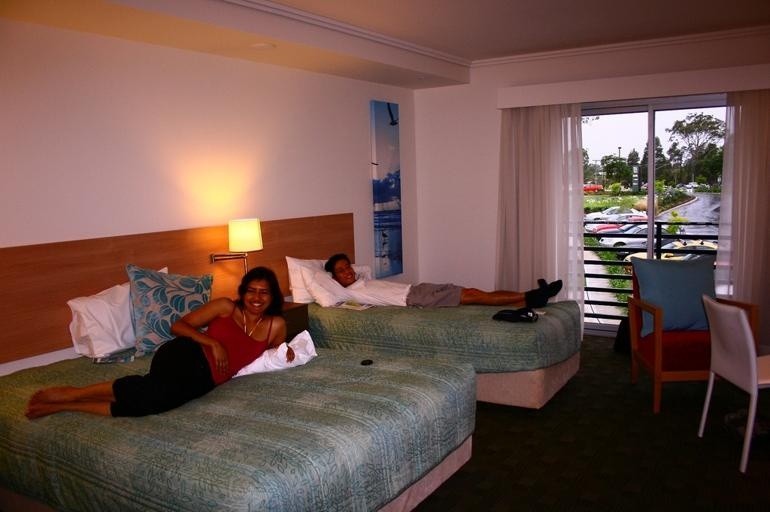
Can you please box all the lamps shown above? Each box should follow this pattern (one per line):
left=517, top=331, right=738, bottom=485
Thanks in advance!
left=210, top=217, right=264, bottom=273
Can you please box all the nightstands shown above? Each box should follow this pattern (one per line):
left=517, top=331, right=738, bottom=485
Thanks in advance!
left=282, top=300, right=309, bottom=341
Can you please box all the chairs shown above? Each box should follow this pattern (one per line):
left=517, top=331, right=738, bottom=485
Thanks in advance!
left=625, top=264, right=770, bottom=472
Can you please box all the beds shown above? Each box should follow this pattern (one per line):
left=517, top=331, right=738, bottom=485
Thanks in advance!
left=0, top=341, right=477, bottom=512
left=284, top=283, right=584, bottom=413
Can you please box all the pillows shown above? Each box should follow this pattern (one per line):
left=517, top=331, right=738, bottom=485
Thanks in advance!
left=630, top=255, right=718, bottom=338
left=67, top=262, right=213, bottom=358
left=283, top=252, right=359, bottom=309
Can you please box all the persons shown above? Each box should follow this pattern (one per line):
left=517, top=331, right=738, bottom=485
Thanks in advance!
left=23, top=266, right=295, bottom=420
left=323, top=249, right=564, bottom=309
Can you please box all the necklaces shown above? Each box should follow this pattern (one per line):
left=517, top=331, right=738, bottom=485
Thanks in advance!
left=243, top=310, right=262, bottom=338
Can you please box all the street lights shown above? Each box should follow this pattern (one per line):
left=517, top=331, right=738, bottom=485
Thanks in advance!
left=618, top=146, right=621, bottom=159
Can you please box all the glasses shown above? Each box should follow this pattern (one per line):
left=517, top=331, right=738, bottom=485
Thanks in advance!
left=527, top=311, right=538, bottom=321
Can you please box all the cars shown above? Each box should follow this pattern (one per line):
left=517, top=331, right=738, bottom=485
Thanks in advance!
left=582, top=180, right=632, bottom=195
left=584, top=206, right=718, bottom=273
left=641, top=181, right=710, bottom=192
